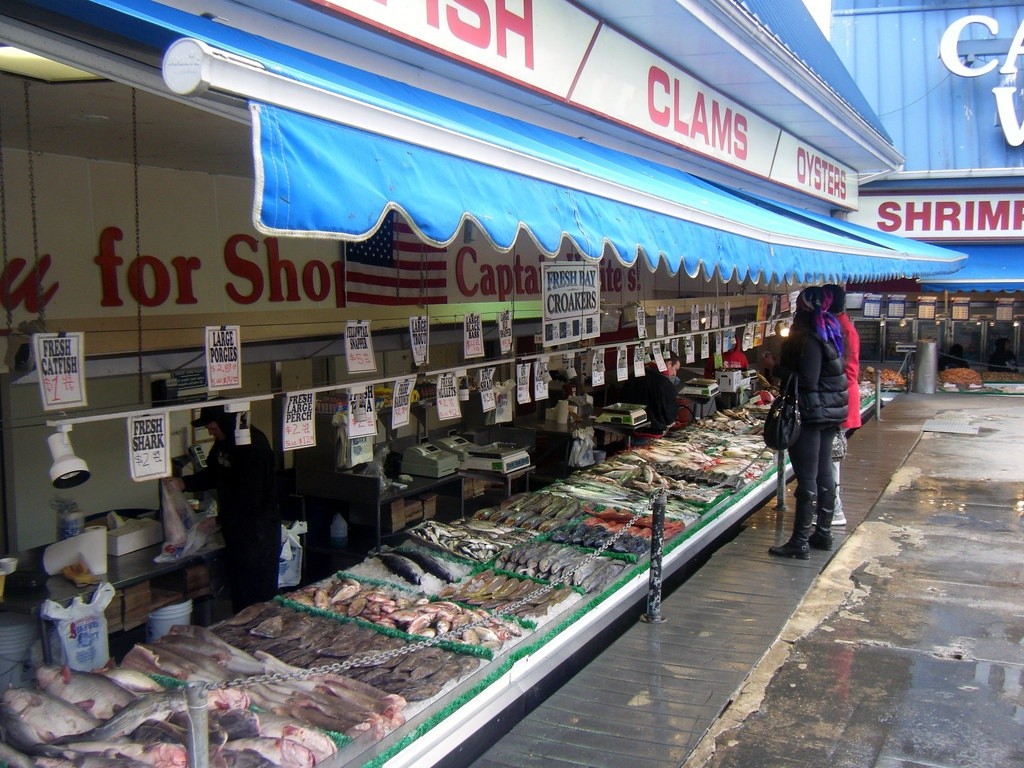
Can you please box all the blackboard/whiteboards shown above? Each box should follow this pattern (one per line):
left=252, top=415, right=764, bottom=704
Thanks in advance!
left=789, top=290, right=799, bottom=313
left=886, top=294, right=907, bottom=318
left=951, top=297, right=970, bottom=321
left=994, top=297, right=1015, bottom=323
left=917, top=296, right=937, bottom=320
left=863, top=294, right=882, bottom=317
left=780, top=294, right=790, bottom=312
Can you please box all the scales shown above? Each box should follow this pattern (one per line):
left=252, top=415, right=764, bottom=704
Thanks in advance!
left=677, top=378, right=720, bottom=397
left=464, top=442, right=531, bottom=474
left=594, top=402, right=649, bottom=426
left=880, top=342, right=917, bottom=393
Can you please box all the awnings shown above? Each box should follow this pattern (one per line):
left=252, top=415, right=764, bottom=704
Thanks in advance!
left=917, top=246, right=1024, bottom=294
left=1, top=1, right=970, bottom=282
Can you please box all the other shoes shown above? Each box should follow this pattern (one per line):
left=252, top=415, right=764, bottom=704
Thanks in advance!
left=812, top=512, right=846, bottom=525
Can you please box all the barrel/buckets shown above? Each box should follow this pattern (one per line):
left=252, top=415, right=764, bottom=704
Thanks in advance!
left=0, top=613, right=38, bottom=696
left=594, top=450, right=606, bottom=464
left=146, top=598, right=193, bottom=643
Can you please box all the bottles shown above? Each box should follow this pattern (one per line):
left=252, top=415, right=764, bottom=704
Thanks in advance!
left=331, top=514, right=347, bottom=547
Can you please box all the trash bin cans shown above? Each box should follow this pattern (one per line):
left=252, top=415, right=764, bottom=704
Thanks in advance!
left=916, top=339, right=938, bottom=395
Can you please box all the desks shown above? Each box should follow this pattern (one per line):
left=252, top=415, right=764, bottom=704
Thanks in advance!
left=329, top=458, right=537, bottom=563
left=498, top=373, right=759, bottom=483
left=20, top=535, right=225, bottom=669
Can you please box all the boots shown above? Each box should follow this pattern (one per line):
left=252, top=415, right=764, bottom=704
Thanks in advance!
left=809, top=483, right=837, bottom=552
left=770, top=485, right=816, bottom=559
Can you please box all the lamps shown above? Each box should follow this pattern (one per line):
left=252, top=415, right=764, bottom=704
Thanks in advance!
left=538, top=357, right=553, bottom=383
left=744, top=325, right=751, bottom=339
left=683, top=336, right=693, bottom=355
left=595, top=349, right=605, bottom=371
left=45, top=424, right=90, bottom=490
left=347, top=387, right=368, bottom=422
left=728, top=328, right=737, bottom=345
left=769, top=323, right=776, bottom=337
left=755, top=325, right=761, bottom=339
left=778, top=320, right=790, bottom=337
left=224, top=401, right=252, bottom=445
left=899, top=320, right=907, bottom=327
left=660, top=340, right=670, bottom=359
left=880, top=321, right=885, bottom=326
left=564, top=353, right=578, bottom=380
left=617, top=345, right=627, bottom=368
left=990, top=321, right=996, bottom=326
left=642, top=342, right=651, bottom=364
left=1013, top=321, right=1019, bottom=327
left=455, top=370, right=469, bottom=401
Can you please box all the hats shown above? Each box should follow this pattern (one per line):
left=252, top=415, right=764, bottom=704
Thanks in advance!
left=796, top=286, right=825, bottom=319
left=822, top=284, right=844, bottom=306
left=191, top=396, right=238, bottom=439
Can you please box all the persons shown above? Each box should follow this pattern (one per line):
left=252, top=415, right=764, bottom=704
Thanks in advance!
left=627, top=351, right=680, bottom=448
left=769, top=290, right=848, bottom=558
left=706, top=338, right=749, bottom=381
left=810, top=284, right=860, bottom=524
left=169, top=398, right=282, bottom=613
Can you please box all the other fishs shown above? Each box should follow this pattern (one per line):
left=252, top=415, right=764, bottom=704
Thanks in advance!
left=162, top=498, right=216, bottom=553
left=344, top=409, right=777, bottom=636
left=0, top=579, right=522, bottom=768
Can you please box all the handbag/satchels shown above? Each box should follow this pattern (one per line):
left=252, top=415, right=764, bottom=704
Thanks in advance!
left=763, top=371, right=801, bottom=450
left=161, top=479, right=199, bottom=546
left=832, top=426, right=848, bottom=462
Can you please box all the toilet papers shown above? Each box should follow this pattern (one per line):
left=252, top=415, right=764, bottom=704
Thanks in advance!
left=557, top=400, right=568, bottom=424
left=43, top=525, right=107, bottom=576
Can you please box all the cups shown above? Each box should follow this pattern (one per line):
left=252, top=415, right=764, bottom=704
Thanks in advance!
left=187, top=499, right=199, bottom=510
left=0, top=569, right=6, bottom=596
left=0, top=558, right=18, bottom=575
